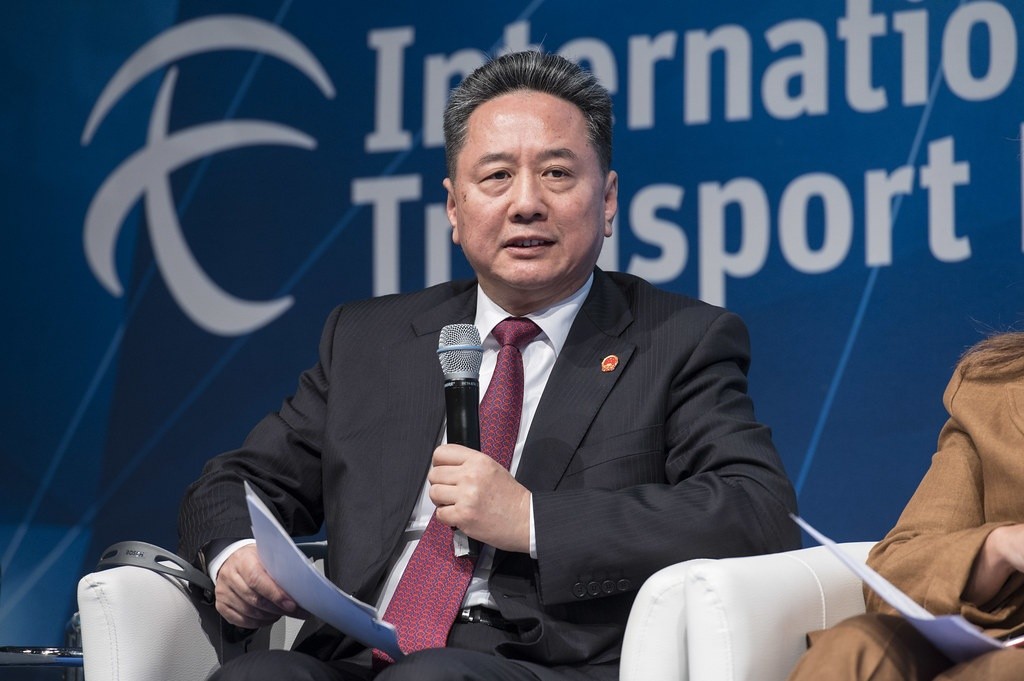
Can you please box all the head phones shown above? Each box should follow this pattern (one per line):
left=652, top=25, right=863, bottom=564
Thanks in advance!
left=96, top=541, right=260, bottom=643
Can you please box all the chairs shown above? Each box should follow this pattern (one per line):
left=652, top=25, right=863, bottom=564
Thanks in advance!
left=78, top=540, right=718, bottom=681
left=684, top=541, right=876, bottom=681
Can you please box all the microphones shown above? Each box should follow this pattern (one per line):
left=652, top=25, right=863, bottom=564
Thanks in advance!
left=438, top=323, right=483, bottom=529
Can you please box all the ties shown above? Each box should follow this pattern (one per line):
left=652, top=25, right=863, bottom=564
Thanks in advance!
left=373, top=316, right=543, bottom=663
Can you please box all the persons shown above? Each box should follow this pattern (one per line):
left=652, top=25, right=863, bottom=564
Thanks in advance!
left=787, top=331, right=1024, bottom=681
left=177, top=50, right=803, bottom=681
